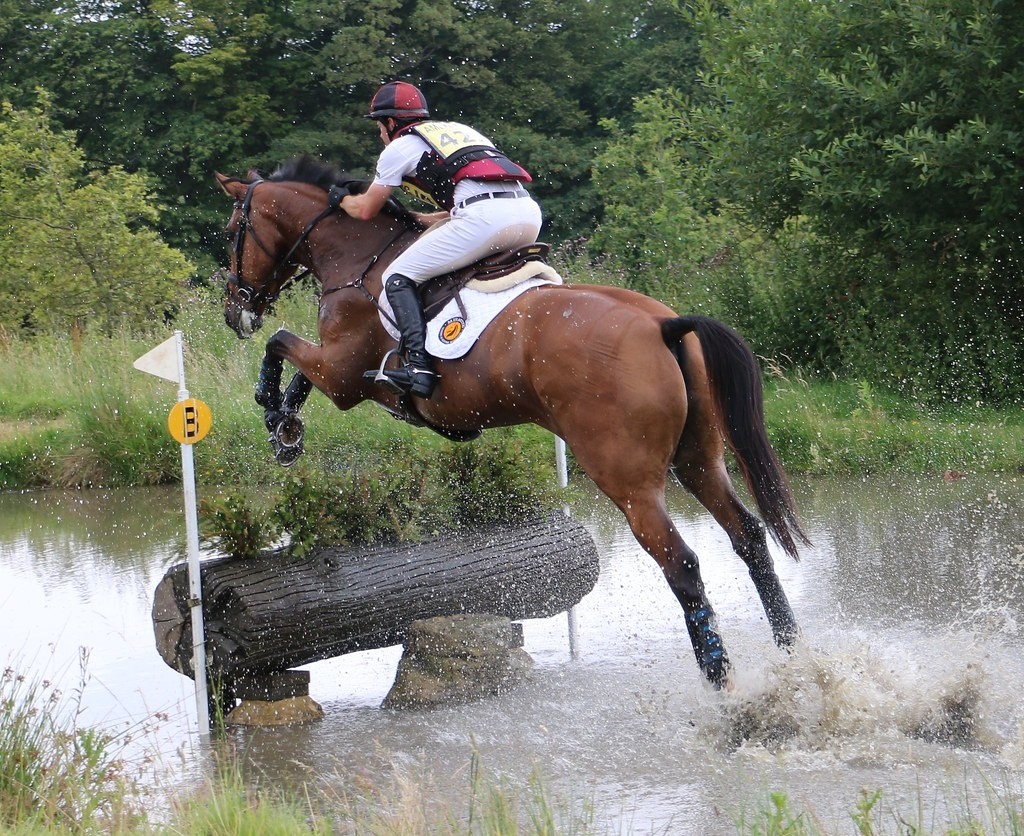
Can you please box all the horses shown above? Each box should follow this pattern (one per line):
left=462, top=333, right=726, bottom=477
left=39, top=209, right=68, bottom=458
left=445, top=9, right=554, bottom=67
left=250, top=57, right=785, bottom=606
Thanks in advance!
left=214, top=154, right=816, bottom=700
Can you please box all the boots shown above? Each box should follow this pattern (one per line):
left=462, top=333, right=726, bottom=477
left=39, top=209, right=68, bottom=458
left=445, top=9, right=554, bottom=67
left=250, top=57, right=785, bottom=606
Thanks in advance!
left=363, top=270, right=435, bottom=398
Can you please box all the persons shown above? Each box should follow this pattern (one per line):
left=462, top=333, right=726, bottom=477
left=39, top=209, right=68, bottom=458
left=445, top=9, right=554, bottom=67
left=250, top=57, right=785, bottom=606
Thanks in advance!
left=327, top=78, right=547, bottom=395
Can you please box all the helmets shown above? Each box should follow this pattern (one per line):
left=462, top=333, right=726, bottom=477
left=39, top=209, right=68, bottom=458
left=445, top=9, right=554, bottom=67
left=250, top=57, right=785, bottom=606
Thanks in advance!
left=363, top=82, right=431, bottom=119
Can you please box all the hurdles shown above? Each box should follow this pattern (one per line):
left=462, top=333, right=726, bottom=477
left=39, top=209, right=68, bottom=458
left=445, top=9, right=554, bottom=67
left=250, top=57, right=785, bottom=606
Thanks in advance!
left=132, top=328, right=602, bottom=735
